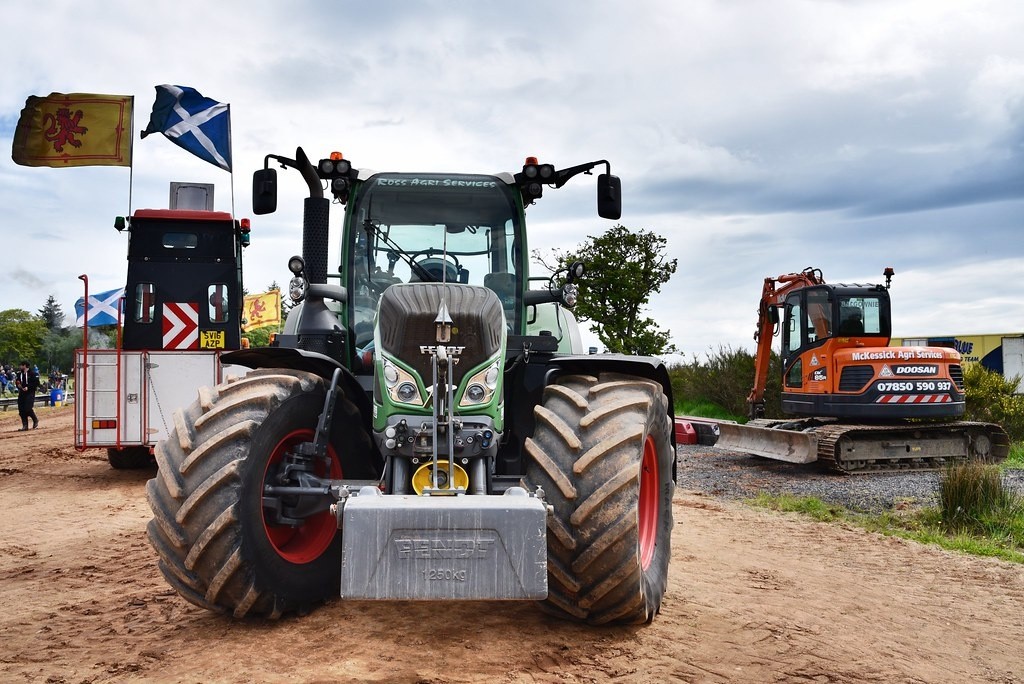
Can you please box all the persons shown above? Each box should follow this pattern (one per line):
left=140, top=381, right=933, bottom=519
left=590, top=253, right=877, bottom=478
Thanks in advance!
left=0, top=363, right=62, bottom=397
left=15, top=360, right=39, bottom=431
left=840, top=308, right=863, bottom=333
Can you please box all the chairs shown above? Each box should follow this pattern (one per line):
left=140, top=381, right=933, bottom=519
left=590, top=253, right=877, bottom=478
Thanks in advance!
left=838, top=306, right=865, bottom=338
left=409, top=248, right=461, bottom=283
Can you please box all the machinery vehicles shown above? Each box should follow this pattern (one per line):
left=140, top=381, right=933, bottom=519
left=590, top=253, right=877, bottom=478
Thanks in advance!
left=708, top=265, right=1012, bottom=478
left=73, top=181, right=250, bottom=470
left=145, top=146, right=720, bottom=630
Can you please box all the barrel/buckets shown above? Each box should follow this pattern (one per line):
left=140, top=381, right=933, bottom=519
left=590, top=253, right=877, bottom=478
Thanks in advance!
left=55, top=401, right=61, bottom=407
left=51, top=388, right=63, bottom=406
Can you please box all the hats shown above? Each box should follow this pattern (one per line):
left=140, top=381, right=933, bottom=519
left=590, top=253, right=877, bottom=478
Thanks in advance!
left=18, top=361, right=30, bottom=368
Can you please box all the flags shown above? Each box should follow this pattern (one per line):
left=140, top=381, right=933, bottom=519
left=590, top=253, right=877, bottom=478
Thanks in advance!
left=75, top=288, right=126, bottom=328
left=138, top=83, right=231, bottom=172
left=12, top=91, right=132, bottom=169
left=240, top=289, right=282, bottom=332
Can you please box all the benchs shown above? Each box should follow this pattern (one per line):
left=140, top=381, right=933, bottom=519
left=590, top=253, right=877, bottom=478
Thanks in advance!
left=0, top=393, right=75, bottom=412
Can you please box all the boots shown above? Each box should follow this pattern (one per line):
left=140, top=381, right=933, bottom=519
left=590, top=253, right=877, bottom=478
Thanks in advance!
left=18, top=421, right=29, bottom=431
left=32, top=417, right=38, bottom=428
